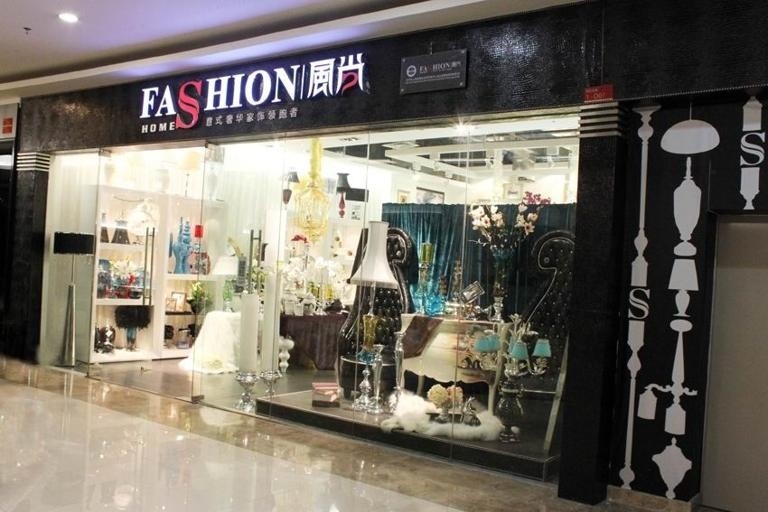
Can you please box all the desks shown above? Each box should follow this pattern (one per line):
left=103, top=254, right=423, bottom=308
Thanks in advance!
left=196, top=308, right=348, bottom=374
left=395, top=310, right=500, bottom=415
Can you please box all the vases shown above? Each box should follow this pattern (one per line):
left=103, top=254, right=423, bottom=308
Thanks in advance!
left=412, top=242, right=448, bottom=314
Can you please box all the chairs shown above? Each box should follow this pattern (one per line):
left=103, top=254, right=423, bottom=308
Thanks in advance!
left=494, top=229, right=575, bottom=401
left=337, top=228, right=414, bottom=402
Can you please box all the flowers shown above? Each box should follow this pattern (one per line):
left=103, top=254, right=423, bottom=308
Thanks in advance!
left=468, top=189, right=551, bottom=297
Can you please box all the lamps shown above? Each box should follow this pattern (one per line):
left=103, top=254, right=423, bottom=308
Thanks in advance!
left=347, top=219, right=401, bottom=361
left=471, top=311, right=552, bottom=442
left=281, top=170, right=299, bottom=206
left=52, top=232, right=96, bottom=368
left=210, top=255, right=241, bottom=312
left=336, top=146, right=351, bottom=216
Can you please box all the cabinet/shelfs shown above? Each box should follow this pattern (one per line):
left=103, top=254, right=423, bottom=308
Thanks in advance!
left=75, top=186, right=231, bottom=363
